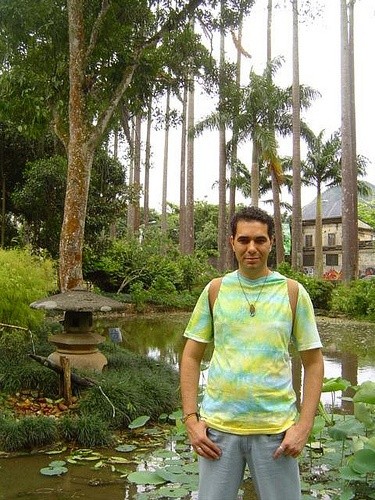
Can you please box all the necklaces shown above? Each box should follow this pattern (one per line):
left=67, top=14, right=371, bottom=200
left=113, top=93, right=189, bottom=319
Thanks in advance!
left=237, top=269, right=269, bottom=318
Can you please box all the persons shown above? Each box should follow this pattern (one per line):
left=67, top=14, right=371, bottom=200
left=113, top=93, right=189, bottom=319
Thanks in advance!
left=181, top=208, right=324, bottom=499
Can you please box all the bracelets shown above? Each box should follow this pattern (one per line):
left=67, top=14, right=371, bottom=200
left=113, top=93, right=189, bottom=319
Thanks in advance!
left=180, top=413, right=198, bottom=424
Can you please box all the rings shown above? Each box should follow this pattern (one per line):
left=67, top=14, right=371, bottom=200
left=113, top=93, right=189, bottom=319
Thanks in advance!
left=196, top=447, right=203, bottom=451
left=278, top=444, right=285, bottom=452
